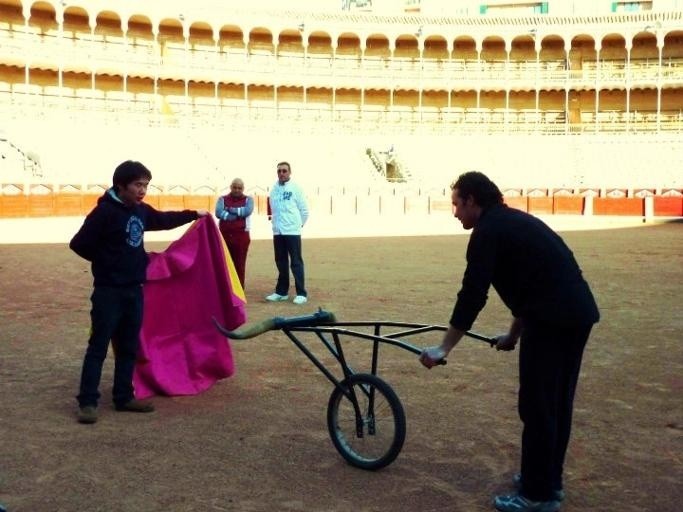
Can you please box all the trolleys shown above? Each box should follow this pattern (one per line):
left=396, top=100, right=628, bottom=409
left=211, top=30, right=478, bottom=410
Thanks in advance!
left=204, top=298, right=514, bottom=472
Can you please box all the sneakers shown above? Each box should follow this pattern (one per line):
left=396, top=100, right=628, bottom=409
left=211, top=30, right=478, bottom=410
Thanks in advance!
left=493, top=491, right=562, bottom=512
left=265, top=292, right=289, bottom=301
left=76, top=403, right=99, bottom=424
left=512, top=472, right=566, bottom=500
left=111, top=394, right=155, bottom=413
left=292, top=295, right=307, bottom=304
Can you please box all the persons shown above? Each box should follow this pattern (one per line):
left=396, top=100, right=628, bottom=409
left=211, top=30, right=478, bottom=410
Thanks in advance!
left=213, top=177, right=255, bottom=290
left=67, top=160, right=210, bottom=424
left=418, top=169, right=601, bottom=512
left=264, top=161, right=309, bottom=305
